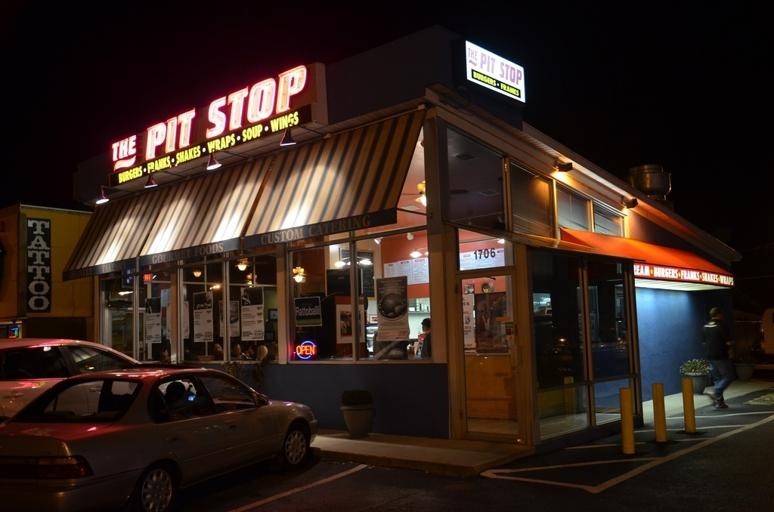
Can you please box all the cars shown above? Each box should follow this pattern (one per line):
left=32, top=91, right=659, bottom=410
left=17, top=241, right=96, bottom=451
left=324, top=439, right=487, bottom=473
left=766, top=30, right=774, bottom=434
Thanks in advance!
left=1, top=364, right=320, bottom=510
left=0, top=337, right=167, bottom=429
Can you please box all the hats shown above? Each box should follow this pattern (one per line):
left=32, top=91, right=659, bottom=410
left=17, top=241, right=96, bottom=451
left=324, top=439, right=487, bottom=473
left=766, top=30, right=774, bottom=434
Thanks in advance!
left=421, top=318, right=430, bottom=326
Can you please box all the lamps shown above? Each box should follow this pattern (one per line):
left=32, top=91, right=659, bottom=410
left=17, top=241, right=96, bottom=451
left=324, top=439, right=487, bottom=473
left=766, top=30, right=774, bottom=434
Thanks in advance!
left=92, top=186, right=112, bottom=208
left=373, top=232, right=415, bottom=245
left=621, top=196, right=638, bottom=208
left=141, top=165, right=163, bottom=193
left=552, top=159, right=572, bottom=172
left=236, top=255, right=249, bottom=272
left=279, top=129, right=298, bottom=149
left=205, top=148, right=225, bottom=173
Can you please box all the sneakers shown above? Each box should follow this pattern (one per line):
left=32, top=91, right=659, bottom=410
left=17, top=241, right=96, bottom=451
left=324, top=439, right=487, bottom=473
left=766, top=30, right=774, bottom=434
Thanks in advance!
left=709, top=393, right=728, bottom=408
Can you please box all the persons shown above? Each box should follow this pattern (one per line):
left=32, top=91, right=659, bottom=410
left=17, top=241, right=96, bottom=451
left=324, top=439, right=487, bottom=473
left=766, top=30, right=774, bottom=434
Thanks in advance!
left=183, top=338, right=198, bottom=361
left=415, top=317, right=430, bottom=358
left=165, top=381, right=187, bottom=405
left=230, top=342, right=243, bottom=359
left=212, top=343, right=222, bottom=355
left=701, top=307, right=736, bottom=408
left=240, top=343, right=256, bottom=360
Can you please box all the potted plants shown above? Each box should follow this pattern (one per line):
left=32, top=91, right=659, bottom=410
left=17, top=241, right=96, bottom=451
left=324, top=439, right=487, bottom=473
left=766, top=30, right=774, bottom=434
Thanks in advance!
left=680, top=358, right=713, bottom=394
left=340, top=388, right=377, bottom=435
left=733, top=350, right=758, bottom=380
left=212, top=359, right=265, bottom=404
